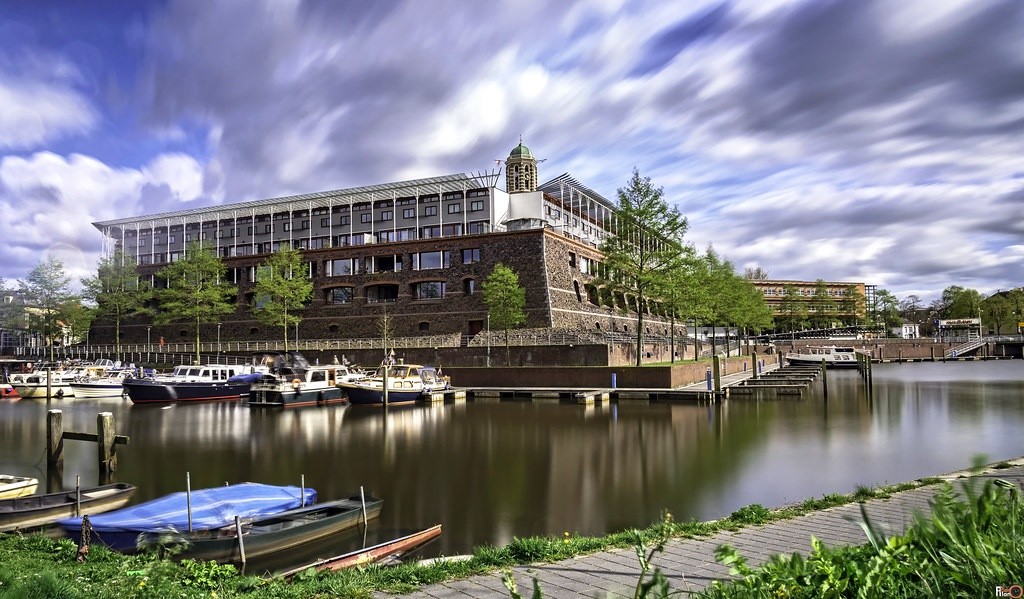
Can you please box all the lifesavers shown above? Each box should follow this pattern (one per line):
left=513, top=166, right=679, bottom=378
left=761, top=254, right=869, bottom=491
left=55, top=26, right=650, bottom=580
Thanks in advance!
left=291, top=379, right=301, bottom=389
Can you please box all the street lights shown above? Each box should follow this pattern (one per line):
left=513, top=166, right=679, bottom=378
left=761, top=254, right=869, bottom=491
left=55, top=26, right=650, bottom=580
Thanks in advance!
left=217, top=323, right=221, bottom=363
left=294, top=321, right=300, bottom=352
left=86, top=328, right=91, bottom=359
left=147, top=326, right=151, bottom=362
left=487, top=315, right=490, bottom=366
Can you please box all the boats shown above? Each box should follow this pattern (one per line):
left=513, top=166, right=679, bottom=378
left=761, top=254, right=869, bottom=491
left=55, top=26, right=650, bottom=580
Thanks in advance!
left=336, top=365, right=450, bottom=404
left=0, top=482, right=136, bottom=532
left=0, top=359, right=138, bottom=398
left=249, top=365, right=375, bottom=405
left=57, top=482, right=317, bottom=554
left=785, top=346, right=882, bottom=364
left=135, top=495, right=383, bottom=565
left=121, top=364, right=269, bottom=403
left=0, top=476, right=39, bottom=500
